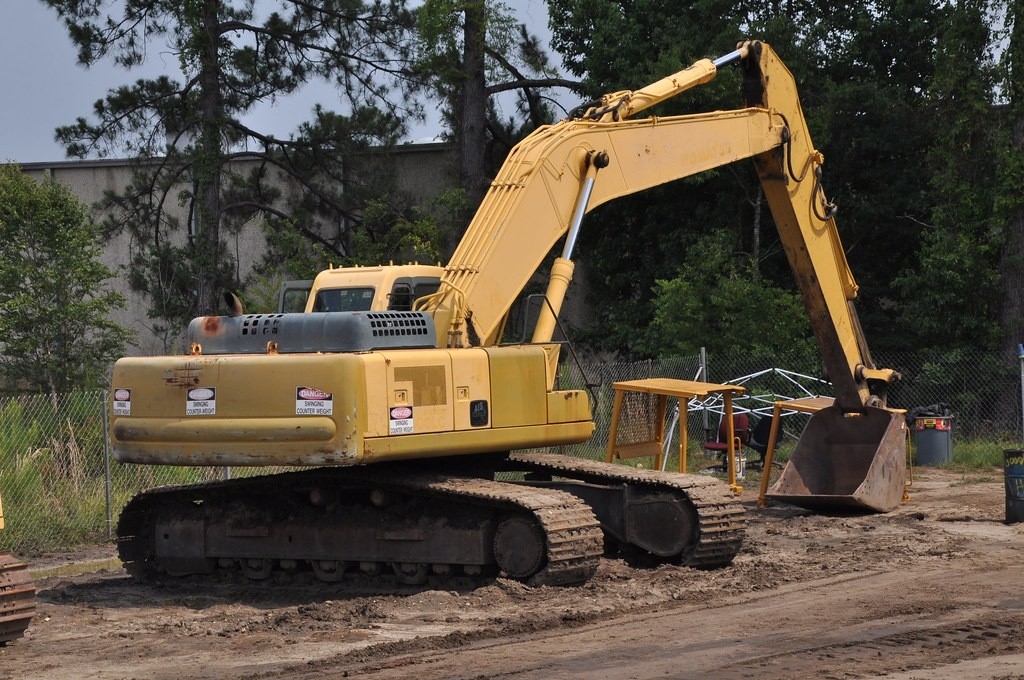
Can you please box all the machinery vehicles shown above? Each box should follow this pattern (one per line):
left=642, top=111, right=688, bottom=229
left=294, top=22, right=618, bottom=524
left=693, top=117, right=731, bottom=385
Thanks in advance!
left=109, top=38, right=911, bottom=590
left=0, top=491, right=37, bottom=646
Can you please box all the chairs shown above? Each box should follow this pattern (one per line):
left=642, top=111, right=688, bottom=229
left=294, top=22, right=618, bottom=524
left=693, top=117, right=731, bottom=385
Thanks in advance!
left=704, top=414, right=749, bottom=473
left=745, top=413, right=786, bottom=471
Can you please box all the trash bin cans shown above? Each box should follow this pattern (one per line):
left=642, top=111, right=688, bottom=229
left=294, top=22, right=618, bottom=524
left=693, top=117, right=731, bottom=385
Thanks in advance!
left=915, top=414, right=954, bottom=466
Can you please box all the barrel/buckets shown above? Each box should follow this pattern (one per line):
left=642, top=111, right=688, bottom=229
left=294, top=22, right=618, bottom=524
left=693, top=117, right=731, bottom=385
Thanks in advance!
left=914, top=416, right=954, bottom=467
left=1002, top=449, right=1024, bottom=525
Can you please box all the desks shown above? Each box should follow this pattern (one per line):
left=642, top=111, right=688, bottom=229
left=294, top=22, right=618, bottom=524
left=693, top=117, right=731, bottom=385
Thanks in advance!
left=605, top=378, right=743, bottom=495
left=757, top=398, right=835, bottom=508
